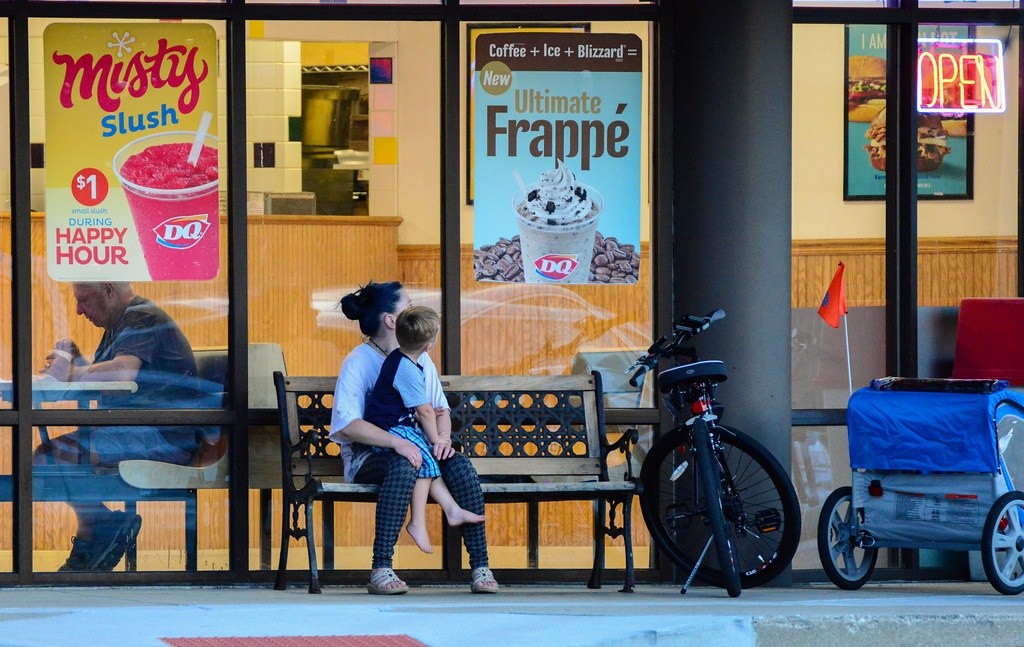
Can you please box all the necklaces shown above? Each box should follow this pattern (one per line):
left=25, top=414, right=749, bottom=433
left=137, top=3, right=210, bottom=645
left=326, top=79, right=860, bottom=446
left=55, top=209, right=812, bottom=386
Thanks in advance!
left=369, top=338, right=388, bottom=357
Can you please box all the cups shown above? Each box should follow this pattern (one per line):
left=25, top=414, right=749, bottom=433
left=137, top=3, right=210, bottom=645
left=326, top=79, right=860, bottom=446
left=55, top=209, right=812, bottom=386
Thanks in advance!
left=111, top=130, right=220, bottom=280
left=53, top=349, right=74, bottom=362
left=511, top=183, right=605, bottom=283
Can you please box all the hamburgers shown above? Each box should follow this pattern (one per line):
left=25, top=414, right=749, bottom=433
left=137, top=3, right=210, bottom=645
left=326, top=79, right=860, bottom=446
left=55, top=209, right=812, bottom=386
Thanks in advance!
left=847, top=55, right=968, bottom=172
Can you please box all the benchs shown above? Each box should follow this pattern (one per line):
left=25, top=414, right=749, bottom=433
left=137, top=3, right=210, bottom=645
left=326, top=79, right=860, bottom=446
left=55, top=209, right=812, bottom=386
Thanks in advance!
left=273, top=369, right=643, bottom=594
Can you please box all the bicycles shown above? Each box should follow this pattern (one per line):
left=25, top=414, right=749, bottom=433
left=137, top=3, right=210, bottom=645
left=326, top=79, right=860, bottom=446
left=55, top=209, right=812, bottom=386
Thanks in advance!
left=623, top=310, right=803, bottom=598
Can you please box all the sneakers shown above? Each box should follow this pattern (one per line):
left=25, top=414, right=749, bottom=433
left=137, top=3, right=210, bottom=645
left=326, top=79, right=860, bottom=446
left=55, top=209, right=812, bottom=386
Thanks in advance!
left=58, top=536, right=95, bottom=571
left=90, top=510, right=142, bottom=572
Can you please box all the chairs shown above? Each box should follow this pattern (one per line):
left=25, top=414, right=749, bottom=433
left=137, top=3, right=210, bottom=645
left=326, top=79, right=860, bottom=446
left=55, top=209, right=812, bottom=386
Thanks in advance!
left=93, top=343, right=287, bottom=571
left=527, top=350, right=663, bottom=568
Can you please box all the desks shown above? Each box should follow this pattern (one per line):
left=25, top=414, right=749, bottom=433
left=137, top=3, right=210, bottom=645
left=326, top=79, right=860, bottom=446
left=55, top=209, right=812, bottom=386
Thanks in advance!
left=0, top=381, right=138, bottom=501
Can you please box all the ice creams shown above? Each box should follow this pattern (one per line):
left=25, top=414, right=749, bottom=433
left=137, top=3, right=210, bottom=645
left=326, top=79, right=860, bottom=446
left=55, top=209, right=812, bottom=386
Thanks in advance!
left=525, top=158, right=593, bottom=225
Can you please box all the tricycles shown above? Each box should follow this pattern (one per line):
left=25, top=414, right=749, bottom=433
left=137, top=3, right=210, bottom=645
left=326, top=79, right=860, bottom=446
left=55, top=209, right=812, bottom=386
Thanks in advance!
left=816, top=376, right=1024, bottom=596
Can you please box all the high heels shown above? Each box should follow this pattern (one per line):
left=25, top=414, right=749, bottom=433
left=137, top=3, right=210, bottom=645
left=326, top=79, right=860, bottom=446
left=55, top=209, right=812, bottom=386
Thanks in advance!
left=366, top=569, right=409, bottom=595
left=470, top=567, right=500, bottom=595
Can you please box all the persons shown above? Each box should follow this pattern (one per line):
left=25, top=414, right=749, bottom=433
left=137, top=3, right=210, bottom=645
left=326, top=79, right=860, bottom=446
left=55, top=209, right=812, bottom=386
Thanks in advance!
left=364, top=306, right=485, bottom=554
left=329, top=278, right=498, bottom=595
left=33, top=282, right=205, bottom=571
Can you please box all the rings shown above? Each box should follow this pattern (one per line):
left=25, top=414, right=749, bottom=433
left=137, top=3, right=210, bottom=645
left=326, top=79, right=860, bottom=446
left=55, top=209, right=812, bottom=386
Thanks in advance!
left=47, top=363, right=49, bottom=368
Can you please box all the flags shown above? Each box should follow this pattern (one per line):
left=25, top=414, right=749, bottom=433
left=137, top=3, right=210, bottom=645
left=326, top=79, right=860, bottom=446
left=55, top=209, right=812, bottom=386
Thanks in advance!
left=817, top=261, right=848, bottom=328
